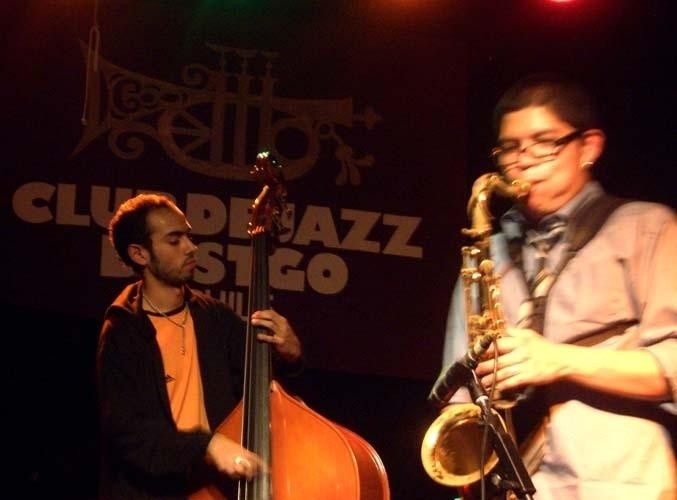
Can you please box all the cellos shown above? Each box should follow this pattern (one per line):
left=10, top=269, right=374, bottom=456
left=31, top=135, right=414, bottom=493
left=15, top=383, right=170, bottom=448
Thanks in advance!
left=187, top=150, right=391, bottom=499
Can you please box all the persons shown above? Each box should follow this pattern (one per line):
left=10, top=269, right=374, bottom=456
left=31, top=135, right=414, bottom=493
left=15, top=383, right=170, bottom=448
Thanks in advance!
left=94, top=191, right=303, bottom=499
left=436, top=72, right=676, bottom=499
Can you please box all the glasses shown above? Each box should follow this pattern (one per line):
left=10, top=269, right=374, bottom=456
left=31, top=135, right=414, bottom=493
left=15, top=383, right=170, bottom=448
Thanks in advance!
left=491, top=131, right=581, bottom=166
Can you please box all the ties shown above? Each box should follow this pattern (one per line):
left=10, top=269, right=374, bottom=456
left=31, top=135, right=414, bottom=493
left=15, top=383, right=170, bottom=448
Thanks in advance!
left=523, top=220, right=565, bottom=334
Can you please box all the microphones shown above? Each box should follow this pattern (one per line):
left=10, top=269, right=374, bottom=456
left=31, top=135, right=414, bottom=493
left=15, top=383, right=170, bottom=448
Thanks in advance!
left=425, top=330, right=500, bottom=413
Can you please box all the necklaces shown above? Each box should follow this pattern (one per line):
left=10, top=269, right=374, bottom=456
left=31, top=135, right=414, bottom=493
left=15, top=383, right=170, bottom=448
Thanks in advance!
left=141, top=293, right=190, bottom=358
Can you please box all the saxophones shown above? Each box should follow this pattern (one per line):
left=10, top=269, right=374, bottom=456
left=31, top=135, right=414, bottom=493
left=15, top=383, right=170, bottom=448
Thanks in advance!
left=420, top=172, right=531, bottom=500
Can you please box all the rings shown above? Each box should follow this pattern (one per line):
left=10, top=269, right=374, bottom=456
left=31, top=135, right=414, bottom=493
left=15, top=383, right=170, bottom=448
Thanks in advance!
left=232, top=456, right=241, bottom=463
